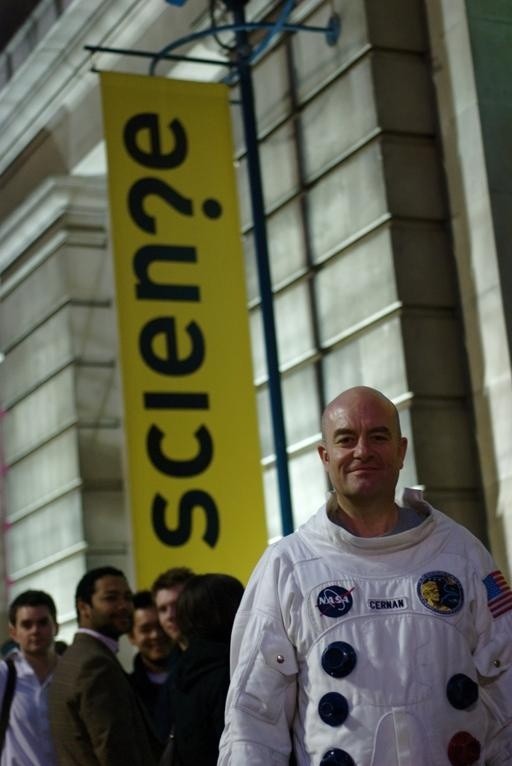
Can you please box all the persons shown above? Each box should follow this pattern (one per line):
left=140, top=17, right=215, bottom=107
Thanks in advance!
left=47, top=564, right=162, bottom=764
left=1, top=589, right=73, bottom=765
left=217, top=384, right=511, bottom=766
left=127, top=567, right=246, bottom=765
